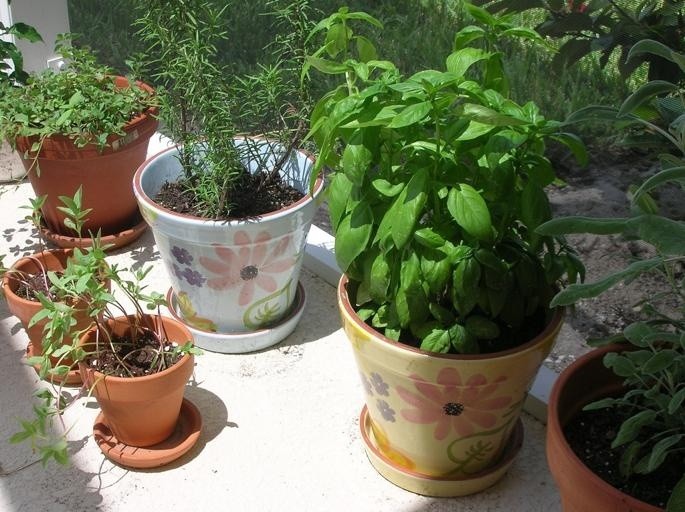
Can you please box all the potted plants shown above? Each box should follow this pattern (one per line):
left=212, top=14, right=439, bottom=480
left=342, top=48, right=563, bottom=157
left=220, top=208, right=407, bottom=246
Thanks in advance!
left=540, top=29, right=684, bottom=512
left=299, top=1, right=587, bottom=502
left=1, top=28, right=162, bottom=254
left=0, top=229, right=204, bottom=471
left=2, top=182, right=114, bottom=387
left=125, top=2, right=327, bottom=358
